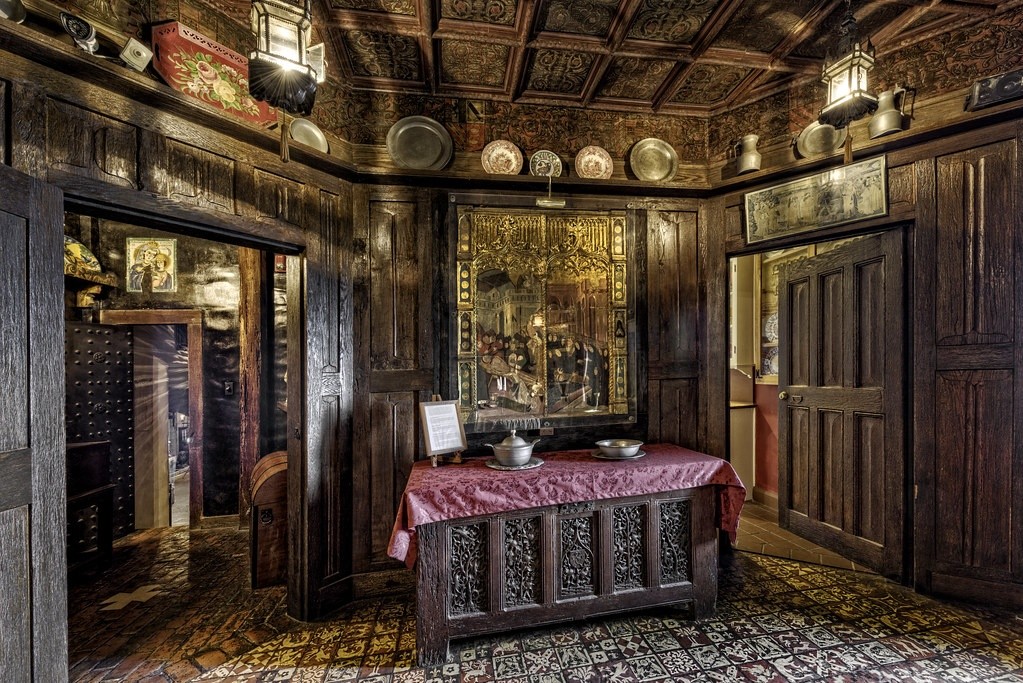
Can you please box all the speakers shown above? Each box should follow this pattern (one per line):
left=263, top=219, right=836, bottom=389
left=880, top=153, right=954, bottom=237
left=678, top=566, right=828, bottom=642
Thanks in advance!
left=963, top=67, right=1023, bottom=112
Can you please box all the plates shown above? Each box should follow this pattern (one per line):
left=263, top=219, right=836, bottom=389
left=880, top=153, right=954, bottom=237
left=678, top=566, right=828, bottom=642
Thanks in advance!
left=630, top=138, right=681, bottom=181
left=575, top=146, right=614, bottom=178
left=387, top=115, right=454, bottom=168
left=484, top=456, right=544, bottom=470
left=592, top=449, right=647, bottom=459
left=289, top=118, right=329, bottom=154
left=481, top=140, right=524, bottom=176
left=530, top=151, right=562, bottom=176
left=797, top=120, right=848, bottom=158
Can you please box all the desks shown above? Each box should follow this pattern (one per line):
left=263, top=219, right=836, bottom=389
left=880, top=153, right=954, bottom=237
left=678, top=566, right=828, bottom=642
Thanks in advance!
left=385, top=442, right=747, bottom=669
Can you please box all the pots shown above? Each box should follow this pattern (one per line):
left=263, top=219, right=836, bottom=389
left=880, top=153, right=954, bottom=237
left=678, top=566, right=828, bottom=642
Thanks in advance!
left=484, top=430, right=541, bottom=465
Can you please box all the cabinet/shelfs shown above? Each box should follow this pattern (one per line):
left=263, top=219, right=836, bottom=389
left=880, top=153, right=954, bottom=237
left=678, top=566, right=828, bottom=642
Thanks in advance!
left=66, top=441, right=117, bottom=588
left=753, top=246, right=815, bottom=385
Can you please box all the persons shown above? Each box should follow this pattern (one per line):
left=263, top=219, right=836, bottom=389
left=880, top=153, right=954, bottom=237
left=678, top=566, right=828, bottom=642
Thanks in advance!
left=471, top=315, right=608, bottom=412
left=130, top=241, right=172, bottom=290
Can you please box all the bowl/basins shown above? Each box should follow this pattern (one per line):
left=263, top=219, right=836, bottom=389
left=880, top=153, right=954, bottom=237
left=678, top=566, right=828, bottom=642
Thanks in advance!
left=595, top=439, right=644, bottom=456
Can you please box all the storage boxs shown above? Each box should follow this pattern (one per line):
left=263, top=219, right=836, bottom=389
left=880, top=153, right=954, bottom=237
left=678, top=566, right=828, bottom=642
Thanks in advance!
left=249, top=452, right=288, bottom=589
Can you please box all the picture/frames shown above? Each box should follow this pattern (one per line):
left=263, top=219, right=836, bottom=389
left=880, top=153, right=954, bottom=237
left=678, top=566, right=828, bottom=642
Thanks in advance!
left=125, top=237, right=178, bottom=293
left=743, top=153, right=889, bottom=247
left=449, top=194, right=638, bottom=435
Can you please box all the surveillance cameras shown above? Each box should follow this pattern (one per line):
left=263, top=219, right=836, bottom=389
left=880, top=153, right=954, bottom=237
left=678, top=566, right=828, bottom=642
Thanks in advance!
left=119, top=36, right=153, bottom=73
left=60, top=12, right=100, bottom=53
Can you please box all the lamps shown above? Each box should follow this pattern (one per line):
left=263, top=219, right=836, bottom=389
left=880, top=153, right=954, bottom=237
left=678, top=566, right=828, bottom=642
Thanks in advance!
left=247, top=1, right=320, bottom=165
left=817, top=2, right=880, bottom=167
left=535, top=176, right=566, bottom=210
